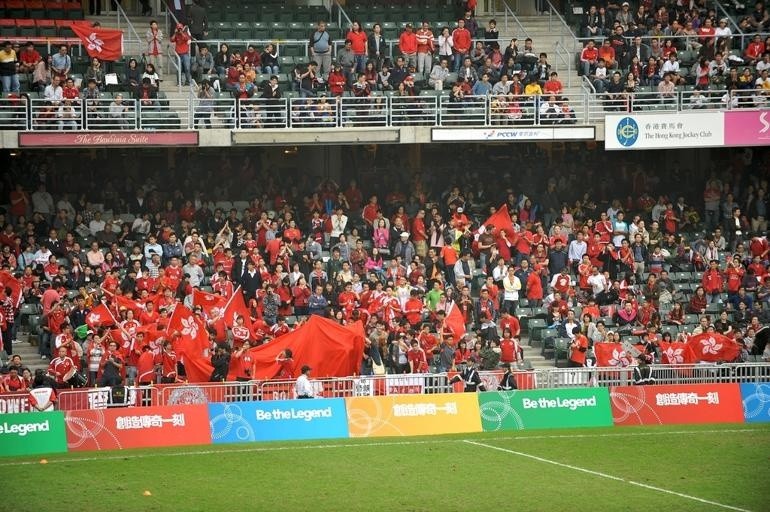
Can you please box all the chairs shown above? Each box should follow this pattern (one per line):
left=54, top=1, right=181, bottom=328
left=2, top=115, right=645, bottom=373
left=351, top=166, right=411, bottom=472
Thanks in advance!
left=511, top=293, right=641, bottom=366
left=1, top=3, right=147, bottom=80
left=180, top=0, right=474, bottom=88
left=647, top=258, right=737, bottom=343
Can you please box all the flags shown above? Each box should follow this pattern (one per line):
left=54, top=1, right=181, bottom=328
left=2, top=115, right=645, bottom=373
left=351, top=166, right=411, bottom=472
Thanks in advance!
left=71, top=22, right=123, bottom=59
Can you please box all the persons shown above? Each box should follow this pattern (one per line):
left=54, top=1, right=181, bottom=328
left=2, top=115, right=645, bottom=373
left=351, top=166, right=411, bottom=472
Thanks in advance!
left=578, top=0, right=769, bottom=112
left=171, top=1, right=578, bottom=124
left=2, top=150, right=769, bottom=411
left=0, top=21, right=163, bottom=128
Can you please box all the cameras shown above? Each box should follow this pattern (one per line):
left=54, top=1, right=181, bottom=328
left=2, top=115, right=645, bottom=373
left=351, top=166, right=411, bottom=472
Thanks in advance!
left=178, top=29, right=180, bottom=32
left=210, top=347, right=218, bottom=352
left=268, top=290, right=271, bottom=295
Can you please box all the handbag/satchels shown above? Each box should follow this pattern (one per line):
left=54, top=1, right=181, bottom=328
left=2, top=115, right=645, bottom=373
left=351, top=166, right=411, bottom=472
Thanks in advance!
left=371, top=359, right=386, bottom=375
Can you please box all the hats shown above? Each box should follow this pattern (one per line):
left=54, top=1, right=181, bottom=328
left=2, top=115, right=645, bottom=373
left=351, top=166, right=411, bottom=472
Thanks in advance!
left=499, top=363, right=511, bottom=368
left=622, top=1, right=630, bottom=7
left=533, top=264, right=545, bottom=270
left=636, top=353, right=646, bottom=361
left=466, top=356, right=475, bottom=362
left=456, top=206, right=465, bottom=214
left=606, top=330, right=615, bottom=336
left=405, top=22, right=413, bottom=28
left=301, top=365, right=312, bottom=371
left=718, top=18, right=728, bottom=24
